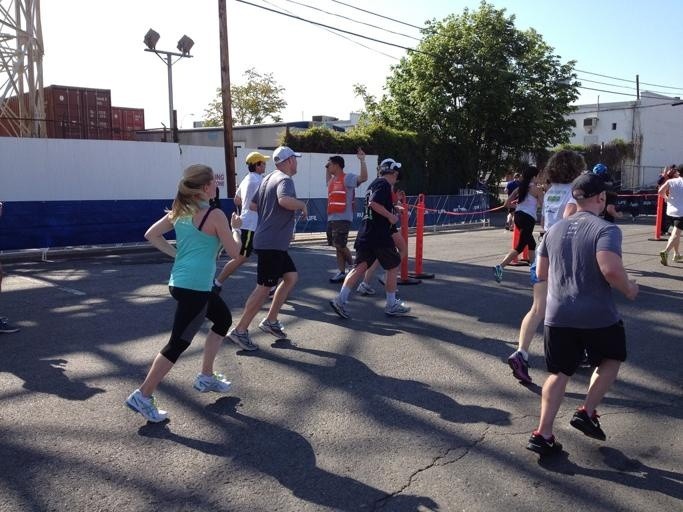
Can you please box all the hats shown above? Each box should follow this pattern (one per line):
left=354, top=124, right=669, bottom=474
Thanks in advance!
left=570, top=174, right=606, bottom=200
left=269, top=147, right=301, bottom=166
left=244, top=153, right=270, bottom=167
left=592, top=163, right=610, bottom=177
left=376, top=157, right=403, bottom=178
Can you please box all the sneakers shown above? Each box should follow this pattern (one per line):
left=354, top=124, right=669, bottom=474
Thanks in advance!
left=672, top=254, right=683, bottom=262
left=507, top=350, right=532, bottom=386
left=525, top=430, right=563, bottom=457
left=259, top=317, right=287, bottom=339
left=356, top=282, right=376, bottom=297
left=268, top=285, right=278, bottom=300
left=384, top=299, right=411, bottom=316
left=123, top=388, right=168, bottom=423
left=330, top=271, right=347, bottom=284
left=211, top=278, right=222, bottom=296
left=492, top=263, right=503, bottom=283
left=660, top=251, right=670, bottom=267
left=226, top=327, right=259, bottom=352
left=191, top=371, right=232, bottom=394
left=328, top=295, right=352, bottom=320
left=0, top=315, right=22, bottom=334
left=570, top=406, right=607, bottom=441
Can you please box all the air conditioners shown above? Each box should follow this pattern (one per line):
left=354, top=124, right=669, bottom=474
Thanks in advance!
left=582, top=118, right=596, bottom=128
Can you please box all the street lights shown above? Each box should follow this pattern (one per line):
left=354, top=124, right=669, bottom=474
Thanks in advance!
left=142, top=28, right=195, bottom=141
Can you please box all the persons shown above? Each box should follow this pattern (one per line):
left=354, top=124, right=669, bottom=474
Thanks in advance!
left=592, top=164, right=624, bottom=224
left=525, top=171, right=640, bottom=455
left=492, top=166, right=546, bottom=283
left=325, top=148, right=367, bottom=284
left=212, top=151, right=279, bottom=300
left=329, top=161, right=411, bottom=319
left=503, top=173, right=520, bottom=231
left=507, top=150, right=591, bottom=384
left=356, top=158, right=406, bottom=294
left=658, top=164, right=683, bottom=266
left=123, top=164, right=242, bottom=423
left=656, top=164, right=680, bottom=236
left=226, top=145, right=308, bottom=351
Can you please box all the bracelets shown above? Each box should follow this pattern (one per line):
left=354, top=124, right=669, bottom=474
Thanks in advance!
left=231, top=229, right=242, bottom=236
